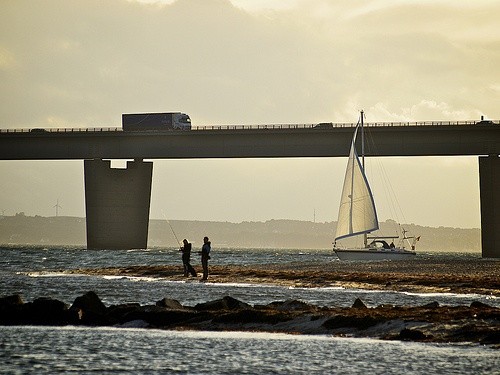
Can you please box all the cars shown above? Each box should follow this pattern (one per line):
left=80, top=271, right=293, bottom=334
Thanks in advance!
left=314, top=123, right=333, bottom=127
left=31, top=128, right=47, bottom=132
left=476, top=121, right=494, bottom=124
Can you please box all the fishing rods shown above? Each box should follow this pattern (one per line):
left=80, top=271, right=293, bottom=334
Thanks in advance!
left=166, top=218, right=184, bottom=253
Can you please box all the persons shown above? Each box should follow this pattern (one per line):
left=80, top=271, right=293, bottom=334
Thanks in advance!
left=390, top=242, right=395, bottom=248
left=182, top=239, right=192, bottom=277
left=201, top=237, right=211, bottom=280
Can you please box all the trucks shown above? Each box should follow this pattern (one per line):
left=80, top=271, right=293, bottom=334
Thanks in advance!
left=122, top=112, right=192, bottom=130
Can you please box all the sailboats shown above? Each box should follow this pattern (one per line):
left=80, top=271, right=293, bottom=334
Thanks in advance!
left=332, top=109, right=423, bottom=263
